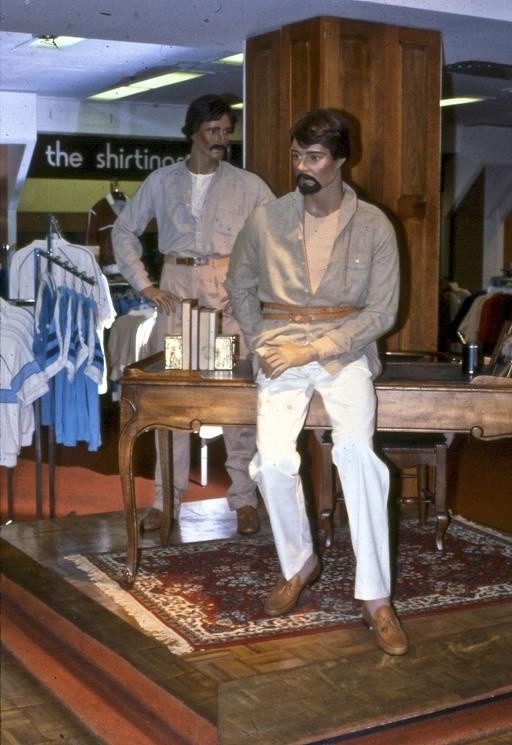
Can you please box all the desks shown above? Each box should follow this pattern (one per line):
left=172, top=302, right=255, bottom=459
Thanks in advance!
left=111, top=346, right=511, bottom=592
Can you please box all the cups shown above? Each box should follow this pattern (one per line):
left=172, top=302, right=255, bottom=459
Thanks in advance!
left=463, top=343, right=480, bottom=373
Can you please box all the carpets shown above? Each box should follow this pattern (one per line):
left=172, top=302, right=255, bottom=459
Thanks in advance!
left=62, top=509, right=510, bottom=659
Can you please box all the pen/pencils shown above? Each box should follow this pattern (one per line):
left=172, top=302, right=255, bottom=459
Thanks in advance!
left=456, top=331, right=468, bottom=345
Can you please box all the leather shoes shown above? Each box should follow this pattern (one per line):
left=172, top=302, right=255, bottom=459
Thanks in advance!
left=362, top=602, right=408, bottom=656
left=140, top=507, right=163, bottom=530
left=236, top=505, right=260, bottom=535
left=264, top=556, right=321, bottom=616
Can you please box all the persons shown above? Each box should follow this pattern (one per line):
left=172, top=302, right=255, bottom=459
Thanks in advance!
left=111, top=93, right=278, bottom=537
left=223, top=108, right=409, bottom=657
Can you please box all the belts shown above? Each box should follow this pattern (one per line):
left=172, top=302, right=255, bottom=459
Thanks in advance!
left=164, top=255, right=229, bottom=267
left=262, top=301, right=362, bottom=323
